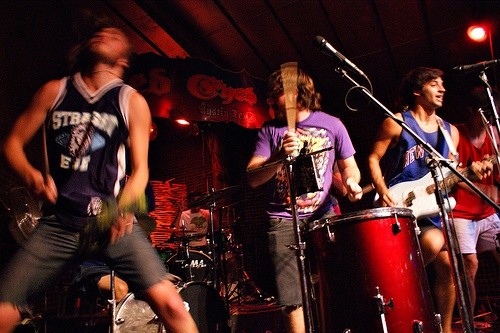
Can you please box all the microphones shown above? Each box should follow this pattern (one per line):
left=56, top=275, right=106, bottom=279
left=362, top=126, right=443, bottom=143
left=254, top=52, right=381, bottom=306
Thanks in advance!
left=453, top=60, right=498, bottom=72
left=314, top=36, right=346, bottom=62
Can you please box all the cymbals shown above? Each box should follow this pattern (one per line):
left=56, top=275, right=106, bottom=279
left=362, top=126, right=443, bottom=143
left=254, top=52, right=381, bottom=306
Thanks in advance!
left=215, top=199, right=249, bottom=213
left=130, top=213, right=157, bottom=236
left=164, top=232, right=203, bottom=244
left=185, top=185, right=241, bottom=208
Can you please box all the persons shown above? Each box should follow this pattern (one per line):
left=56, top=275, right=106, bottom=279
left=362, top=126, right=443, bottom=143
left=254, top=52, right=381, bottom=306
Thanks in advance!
left=239, top=68, right=364, bottom=333
left=64, top=260, right=128, bottom=333
left=177, top=191, right=212, bottom=246
left=0, top=18, right=200, bottom=333
left=367, top=64, right=460, bottom=333
left=452, top=86, right=500, bottom=333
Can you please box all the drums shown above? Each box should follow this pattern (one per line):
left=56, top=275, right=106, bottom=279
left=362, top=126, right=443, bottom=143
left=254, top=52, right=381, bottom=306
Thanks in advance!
left=231, top=304, right=306, bottom=333
left=221, top=244, right=265, bottom=281
left=300, top=207, right=440, bottom=333
left=167, top=251, right=214, bottom=281
left=189, top=238, right=218, bottom=260
left=217, top=232, right=233, bottom=242
left=109, top=281, right=213, bottom=333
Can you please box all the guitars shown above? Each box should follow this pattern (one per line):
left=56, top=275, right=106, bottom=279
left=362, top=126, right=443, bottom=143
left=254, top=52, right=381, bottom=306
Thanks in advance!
left=388, top=154, right=498, bottom=220
